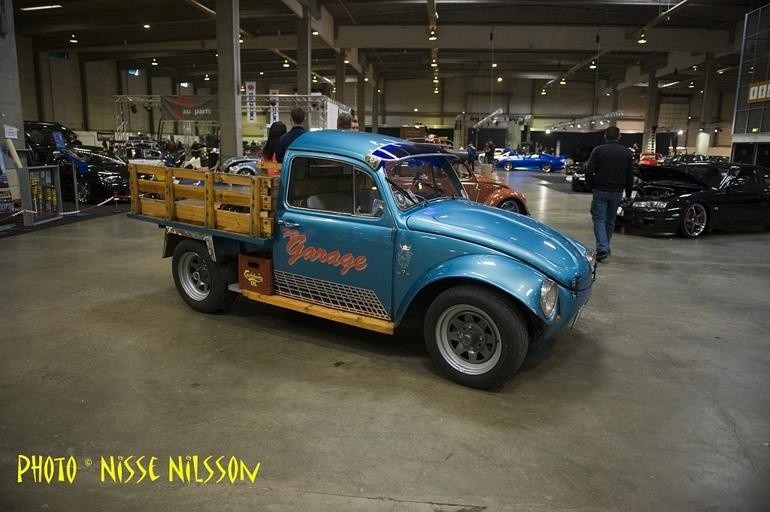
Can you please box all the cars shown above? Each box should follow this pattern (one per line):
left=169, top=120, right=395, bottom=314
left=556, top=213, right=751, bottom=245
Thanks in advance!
left=0, top=117, right=268, bottom=222
left=387, top=153, right=530, bottom=216
left=615, top=162, right=769, bottom=242
left=399, top=137, right=723, bottom=195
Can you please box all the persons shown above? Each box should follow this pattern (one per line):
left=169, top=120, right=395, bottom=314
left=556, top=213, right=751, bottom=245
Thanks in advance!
left=458, top=140, right=555, bottom=171
left=262, top=120, right=286, bottom=173
left=350, top=119, right=360, bottom=131
left=336, top=112, right=351, bottom=130
left=633, top=142, right=641, bottom=164
left=165, top=130, right=256, bottom=156
left=276, top=107, right=306, bottom=166
left=586, top=126, right=634, bottom=263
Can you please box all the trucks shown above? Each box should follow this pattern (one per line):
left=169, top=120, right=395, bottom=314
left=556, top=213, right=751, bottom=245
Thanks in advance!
left=121, top=125, right=601, bottom=390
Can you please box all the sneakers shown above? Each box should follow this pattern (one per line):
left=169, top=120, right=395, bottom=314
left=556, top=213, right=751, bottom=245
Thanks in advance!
left=596, top=251, right=608, bottom=261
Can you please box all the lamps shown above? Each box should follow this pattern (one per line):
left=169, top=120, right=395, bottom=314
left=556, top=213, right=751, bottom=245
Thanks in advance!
left=540, top=34, right=650, bottom=98
left=70, top=33, right=250, bottom=94
left=426, top=27, right=443, bottom=97
left=271, top=27, right=382, bottom=96
